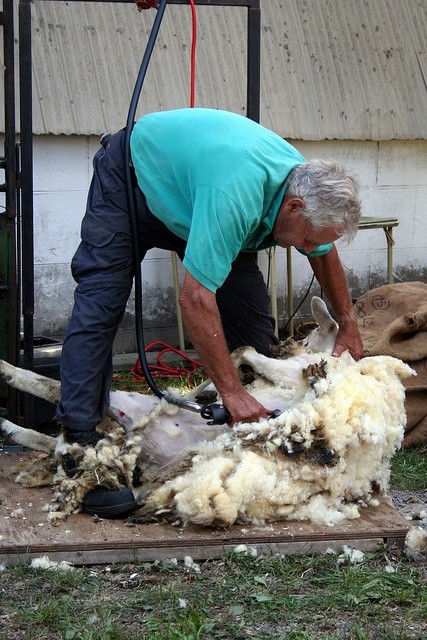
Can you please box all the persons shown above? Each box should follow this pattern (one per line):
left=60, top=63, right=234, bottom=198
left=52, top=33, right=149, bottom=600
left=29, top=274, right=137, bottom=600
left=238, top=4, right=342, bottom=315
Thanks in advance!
left=58, top=107, right=362, bottom=519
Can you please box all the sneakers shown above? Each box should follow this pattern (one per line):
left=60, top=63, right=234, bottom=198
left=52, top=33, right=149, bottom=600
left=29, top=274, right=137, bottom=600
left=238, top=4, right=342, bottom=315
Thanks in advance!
left=64, top=485, right=138, bottom=517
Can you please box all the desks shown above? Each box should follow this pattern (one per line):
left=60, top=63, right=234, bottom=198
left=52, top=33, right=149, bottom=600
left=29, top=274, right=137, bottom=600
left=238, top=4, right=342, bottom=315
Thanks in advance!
left=168, top=214, right=402, bottom=357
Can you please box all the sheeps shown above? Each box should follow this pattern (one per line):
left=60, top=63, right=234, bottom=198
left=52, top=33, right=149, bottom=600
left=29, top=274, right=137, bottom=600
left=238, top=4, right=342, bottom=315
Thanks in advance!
left=0, top=295, right=358, bottom=485
